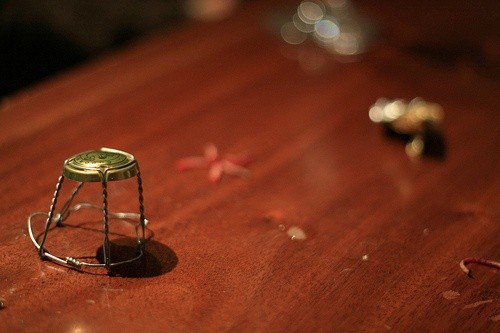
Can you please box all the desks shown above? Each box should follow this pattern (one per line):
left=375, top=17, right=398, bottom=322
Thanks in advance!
left=0, top=0, right=500, bottom=332
